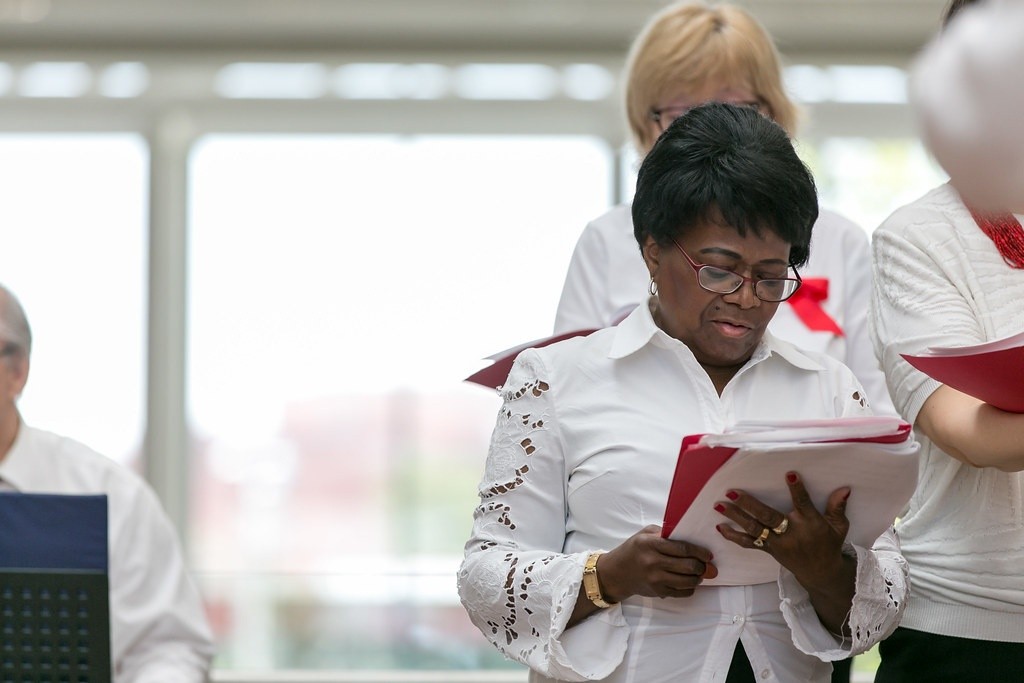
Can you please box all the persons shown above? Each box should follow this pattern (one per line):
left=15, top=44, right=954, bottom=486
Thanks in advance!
left=456, top=0, right=911, bottom=683
left=873, top=0, right=1024, bottom=683
left=0, top=289, right=214, bottom=683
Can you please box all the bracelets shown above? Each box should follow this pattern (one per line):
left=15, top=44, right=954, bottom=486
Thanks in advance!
left=583, top=551, right=620, bottom=609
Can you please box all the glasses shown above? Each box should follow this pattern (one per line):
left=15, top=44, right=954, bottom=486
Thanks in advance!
left=663, top=230, right=803, bottom=303
left=649, top=100, right=766, bottom=133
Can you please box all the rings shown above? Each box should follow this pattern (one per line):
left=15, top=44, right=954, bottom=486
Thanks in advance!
left=753, top=538, right=763, bottom=546
left=759, top=528, right=770, bottom=540
left=773, top=519, right=789, bottom=534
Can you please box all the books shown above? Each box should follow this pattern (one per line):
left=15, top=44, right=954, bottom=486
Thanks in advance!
left=662, top=416, right=921, bottom=586
left=899, top=332, right=1024, bottom=414
left=462, top=303, right=643, bottom=391
